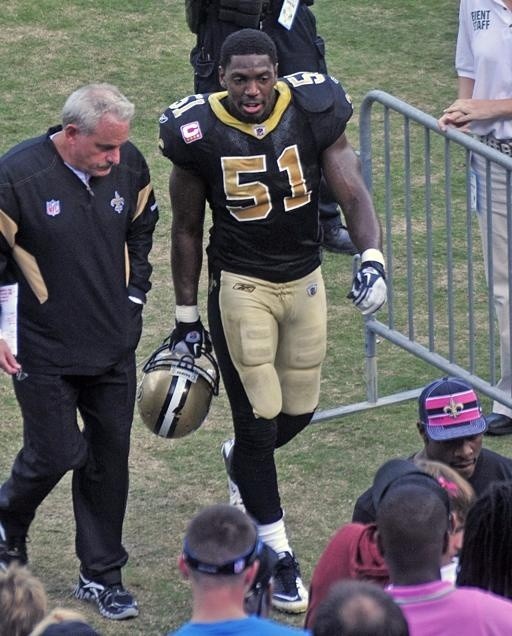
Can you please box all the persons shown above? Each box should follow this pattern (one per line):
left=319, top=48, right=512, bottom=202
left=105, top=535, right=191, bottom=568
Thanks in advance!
left=187, top=0, right=359, bottom=256
left=169, top=504, right=310, bottom=636
left=437, top=0, right=512, bottom=435
left=1, top=558, right=101, bottom=636
left=158, top=27, right=387, bottom=615
left=0, top=82, right=159, bottom=621
left=304, top=460, right=512, bottom=636
left=352, top=377, right=512, bottom=524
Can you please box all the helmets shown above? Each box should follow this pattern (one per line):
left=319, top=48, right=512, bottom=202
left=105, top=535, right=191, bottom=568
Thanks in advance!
left=137, top=337, right=218, bottom=438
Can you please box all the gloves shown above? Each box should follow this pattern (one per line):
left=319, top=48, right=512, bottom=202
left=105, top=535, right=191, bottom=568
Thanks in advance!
left=170, top=305, right=212, bottom=358
left=346, top=249, right=388, bottom=316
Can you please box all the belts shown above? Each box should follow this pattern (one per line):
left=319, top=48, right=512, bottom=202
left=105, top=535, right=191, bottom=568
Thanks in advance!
left=481, top=135, right=512, bottom=157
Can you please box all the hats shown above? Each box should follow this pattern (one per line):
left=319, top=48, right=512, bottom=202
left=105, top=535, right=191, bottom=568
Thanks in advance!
left=372, top=459, right=443, bottom=514
left=419, top=376, right=488, bottom=442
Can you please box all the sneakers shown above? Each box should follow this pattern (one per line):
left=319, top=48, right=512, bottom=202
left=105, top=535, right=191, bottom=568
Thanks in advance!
left=221, top=439, right=247, bottom=514
left=484, top=413, right=512, bottom=435
left=269, top=551, right=309, bottom=614
left=322, top=225, right=358, bottom=253
left=73, top=572, right=139, bottom=619
left=0, top=525, right=32, bottom=573
